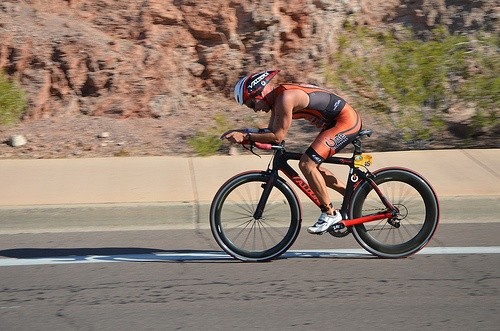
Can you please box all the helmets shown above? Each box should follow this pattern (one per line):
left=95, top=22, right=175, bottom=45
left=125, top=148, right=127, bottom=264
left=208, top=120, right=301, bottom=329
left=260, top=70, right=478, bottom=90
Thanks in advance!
left=234, top=68, right=281, bottom=108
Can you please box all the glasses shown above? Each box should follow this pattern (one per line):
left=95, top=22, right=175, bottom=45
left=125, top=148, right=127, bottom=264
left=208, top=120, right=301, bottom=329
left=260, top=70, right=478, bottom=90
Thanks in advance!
left=246, top=93, right=257, bottom=109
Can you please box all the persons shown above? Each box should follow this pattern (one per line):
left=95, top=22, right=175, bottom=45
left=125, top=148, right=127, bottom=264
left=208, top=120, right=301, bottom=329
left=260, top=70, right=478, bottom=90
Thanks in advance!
left=223, top=70, right=362, bottom=234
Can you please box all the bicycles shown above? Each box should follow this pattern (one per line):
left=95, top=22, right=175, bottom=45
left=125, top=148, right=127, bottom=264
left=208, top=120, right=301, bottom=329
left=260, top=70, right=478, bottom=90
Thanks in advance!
left=210, top=128, right=439, bottom=261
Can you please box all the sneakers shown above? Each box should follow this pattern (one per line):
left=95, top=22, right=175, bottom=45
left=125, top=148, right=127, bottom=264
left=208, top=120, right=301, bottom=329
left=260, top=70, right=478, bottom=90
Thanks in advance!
left=307, top=210, right=343, bottom=234
